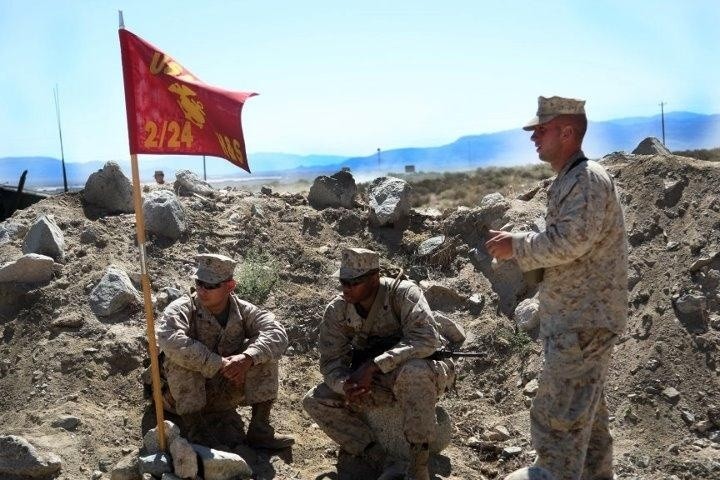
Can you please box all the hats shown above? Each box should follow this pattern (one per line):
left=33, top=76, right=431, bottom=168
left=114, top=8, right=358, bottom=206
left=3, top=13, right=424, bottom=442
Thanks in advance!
left=337, top=248, right=380, bottom=280
left=189, top=253, right=237, bottom=284
left=522, top=95, right=586, bottom=131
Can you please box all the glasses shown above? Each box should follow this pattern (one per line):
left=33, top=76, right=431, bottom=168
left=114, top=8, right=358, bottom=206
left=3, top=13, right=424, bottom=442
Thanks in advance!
left=338, top=269, right=373, bottom=287
left=194, top=278, right=230, bottom=291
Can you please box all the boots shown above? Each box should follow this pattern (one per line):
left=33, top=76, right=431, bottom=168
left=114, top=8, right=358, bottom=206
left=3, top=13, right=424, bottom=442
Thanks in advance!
left=364, top=440, right=408, bottom=480
left=246, top=401, right=295, bottom=450
left=406, top=445, right=431, bottom=480
left=180, top=411, right=217, bottom=447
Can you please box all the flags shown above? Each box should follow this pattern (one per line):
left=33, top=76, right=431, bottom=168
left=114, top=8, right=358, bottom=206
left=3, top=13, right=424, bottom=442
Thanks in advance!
left=119, top=30, right=260, bottom=174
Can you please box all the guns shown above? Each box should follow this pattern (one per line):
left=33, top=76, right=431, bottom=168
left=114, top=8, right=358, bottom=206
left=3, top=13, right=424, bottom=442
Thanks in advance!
left=351, top=336, right=486, bottom=370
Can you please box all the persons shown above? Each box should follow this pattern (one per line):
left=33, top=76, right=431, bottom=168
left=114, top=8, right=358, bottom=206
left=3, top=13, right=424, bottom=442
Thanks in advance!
left=138, top=255, right=295, bottom=450
left=301, top=246, right=457, bottom=480
left=485, top=96, right=628, bottom=480
left=153, top=171, right=169, bottom=185
